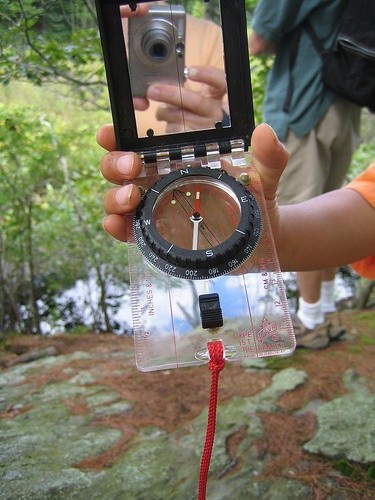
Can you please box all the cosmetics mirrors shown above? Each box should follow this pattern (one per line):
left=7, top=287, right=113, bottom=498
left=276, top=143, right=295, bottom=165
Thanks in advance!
left=95, top=0, right=256, bottom=155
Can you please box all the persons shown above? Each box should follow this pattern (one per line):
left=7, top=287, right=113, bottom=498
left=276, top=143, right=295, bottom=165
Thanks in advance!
left=96, top=123, right=375, bottom=281
left=120, top=0, right=231, bottom=138
left=248, top=0, right=362, bottom=351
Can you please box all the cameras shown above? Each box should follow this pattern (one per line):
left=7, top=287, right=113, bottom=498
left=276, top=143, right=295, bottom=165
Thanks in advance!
left=128, top=4, right=186, bottom=98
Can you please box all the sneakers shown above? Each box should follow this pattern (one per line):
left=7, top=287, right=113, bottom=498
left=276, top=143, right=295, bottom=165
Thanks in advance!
left=256, top=313, right=330, bottom=349
left=324, top=309, right=346, bottom=341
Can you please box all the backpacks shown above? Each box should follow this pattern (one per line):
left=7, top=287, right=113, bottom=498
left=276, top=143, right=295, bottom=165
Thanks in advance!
left=321, top=0, right=375, bottom=114
